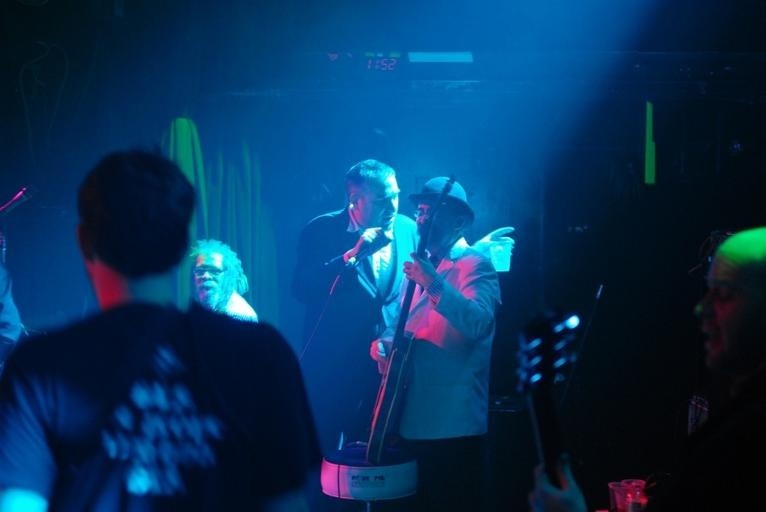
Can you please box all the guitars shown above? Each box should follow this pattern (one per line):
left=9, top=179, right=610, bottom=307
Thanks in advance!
left=364, top=177, right=455, bottom=465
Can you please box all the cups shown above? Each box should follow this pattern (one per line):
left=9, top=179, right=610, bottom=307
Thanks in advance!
left=606, top=477, right=646, bottom=512
left=376, top=340, right=395, bottom=376
left=488, top=241, right=513, bottom=273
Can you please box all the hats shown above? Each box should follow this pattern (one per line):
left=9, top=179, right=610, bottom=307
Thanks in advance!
left=408, top=176, right=474, bottom=222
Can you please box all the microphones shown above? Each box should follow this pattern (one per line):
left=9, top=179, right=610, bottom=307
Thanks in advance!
left=346, top=231, right=394, bottom=269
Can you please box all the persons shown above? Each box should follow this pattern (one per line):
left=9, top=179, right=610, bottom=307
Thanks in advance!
left=185, top=239, right=260, bottom=322
left=0, top=147, right=326, bottom=510
left=369, top=176, right=504, bottom=510
left=528, top=224, right=766, bottom=511
left=296, top=157, right=423, bottom=457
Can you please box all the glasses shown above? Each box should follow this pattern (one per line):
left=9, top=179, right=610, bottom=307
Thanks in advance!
left=411, top=208, right=441, bottom=219
